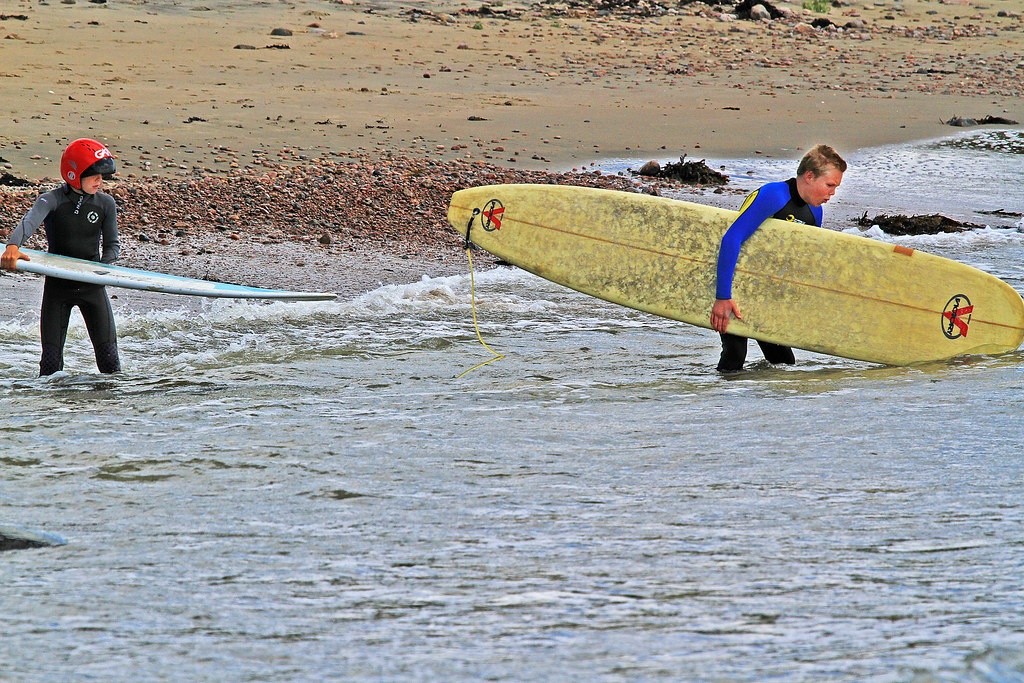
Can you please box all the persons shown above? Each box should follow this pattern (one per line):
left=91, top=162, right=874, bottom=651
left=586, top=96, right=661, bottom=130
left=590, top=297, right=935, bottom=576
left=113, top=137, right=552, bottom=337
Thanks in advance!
left=0, top=138, right=120, bottom=377
left=711, top=144, right=847, bottom=372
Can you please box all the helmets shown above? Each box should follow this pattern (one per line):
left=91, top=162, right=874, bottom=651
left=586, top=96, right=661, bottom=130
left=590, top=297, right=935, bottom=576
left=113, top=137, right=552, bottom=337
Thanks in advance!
left=60, top=138, right=116, bottom=189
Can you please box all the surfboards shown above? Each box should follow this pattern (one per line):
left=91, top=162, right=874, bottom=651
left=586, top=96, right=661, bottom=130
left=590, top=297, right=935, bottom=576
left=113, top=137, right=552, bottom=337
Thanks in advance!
left=446, top=178, right=1024, bottom=366
left=0, top=244, right=338, bottom=305
